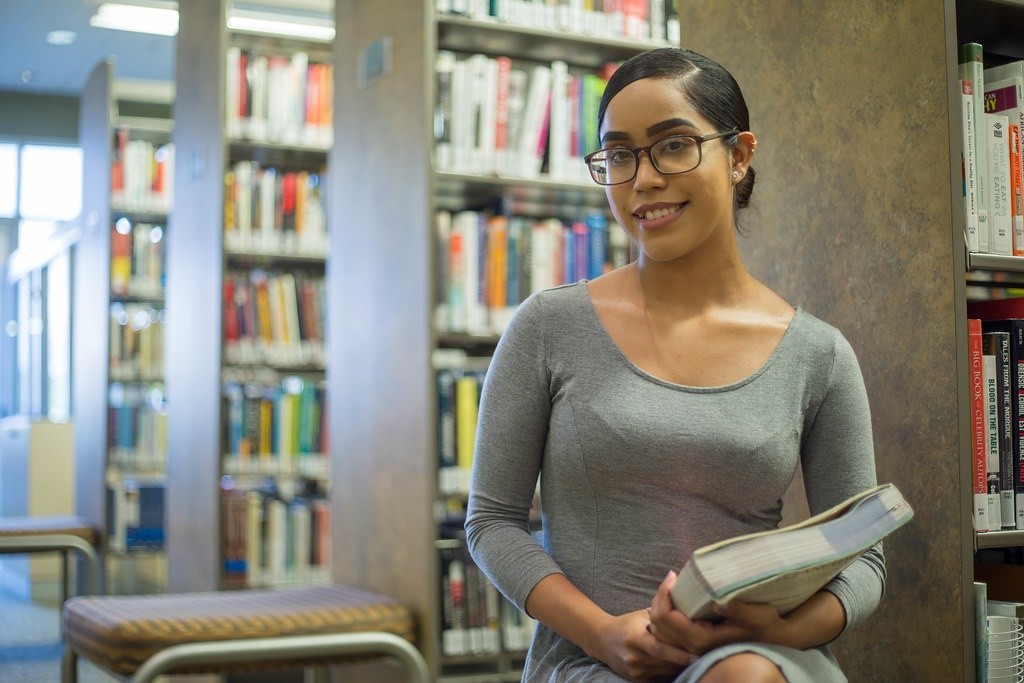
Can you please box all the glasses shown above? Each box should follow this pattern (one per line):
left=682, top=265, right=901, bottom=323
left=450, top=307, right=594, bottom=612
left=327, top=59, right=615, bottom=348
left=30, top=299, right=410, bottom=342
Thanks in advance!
left=584, top=129, right=742, bottom=186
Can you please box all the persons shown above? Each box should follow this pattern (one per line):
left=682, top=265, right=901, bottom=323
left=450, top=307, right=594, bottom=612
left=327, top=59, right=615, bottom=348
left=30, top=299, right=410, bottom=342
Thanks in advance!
left=463, top=49, right=887, bottom=682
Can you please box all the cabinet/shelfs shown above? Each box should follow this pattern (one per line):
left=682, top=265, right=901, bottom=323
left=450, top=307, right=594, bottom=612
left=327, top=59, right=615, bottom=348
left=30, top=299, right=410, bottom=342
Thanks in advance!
left=71, top=0, right=1023, bottom=682
left=0, top=418, right=72, bottom=600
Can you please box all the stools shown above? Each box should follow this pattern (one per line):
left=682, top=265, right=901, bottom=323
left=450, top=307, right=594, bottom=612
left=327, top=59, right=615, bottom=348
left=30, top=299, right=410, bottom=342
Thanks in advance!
left=0, top=514, right=105, bottom=597
left=63, top=580, right=433, bottom=683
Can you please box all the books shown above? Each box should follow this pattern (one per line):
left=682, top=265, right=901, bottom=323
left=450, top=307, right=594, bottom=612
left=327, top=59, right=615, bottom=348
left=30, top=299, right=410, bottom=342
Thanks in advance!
left=106, top=0, right=669, bottom=655
left=668, top=484, right=914, bottom=619
left=959, top=43, right=1023, bottom=536
